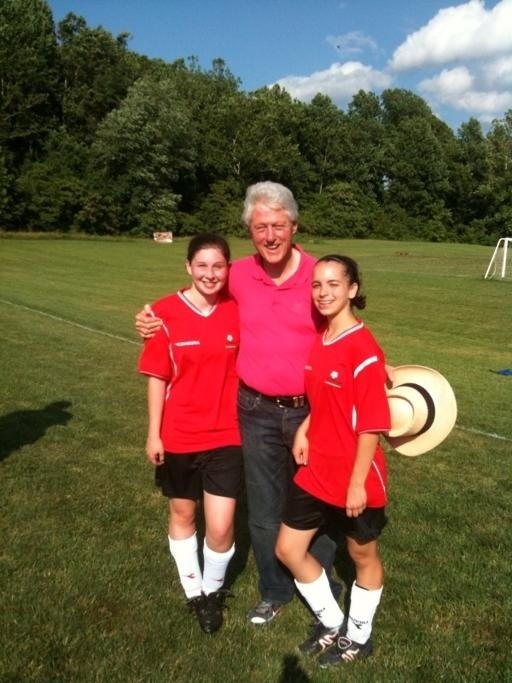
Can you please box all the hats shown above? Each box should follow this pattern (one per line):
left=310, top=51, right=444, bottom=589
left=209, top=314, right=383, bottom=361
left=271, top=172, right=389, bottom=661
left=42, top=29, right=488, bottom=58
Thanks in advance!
left=379, top=362, right=459, bottom=457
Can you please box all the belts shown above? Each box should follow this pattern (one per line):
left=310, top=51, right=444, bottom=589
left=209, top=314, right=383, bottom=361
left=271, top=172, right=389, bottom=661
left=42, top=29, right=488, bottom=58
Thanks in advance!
left=236, top=376, right=309, bottom=409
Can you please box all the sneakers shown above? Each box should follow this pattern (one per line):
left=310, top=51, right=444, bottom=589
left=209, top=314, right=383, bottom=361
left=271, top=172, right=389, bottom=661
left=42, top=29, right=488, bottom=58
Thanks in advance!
left=308, top=583, right=348, bottom=628
left=185, top=586, right=230, bottom=634
left=315, top=627, right=374, bottom=670
left=297, top=614, right=348, bottom=658
left=248, top=596, right=285, bottom=624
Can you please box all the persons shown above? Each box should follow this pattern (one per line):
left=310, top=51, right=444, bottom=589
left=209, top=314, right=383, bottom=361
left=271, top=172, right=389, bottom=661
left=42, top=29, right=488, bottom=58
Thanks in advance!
left=274, top=252, right=392, bottom=669
left=133, top=179, right=398, bottom=627
left=136, top=231, right=241, bottom=636
